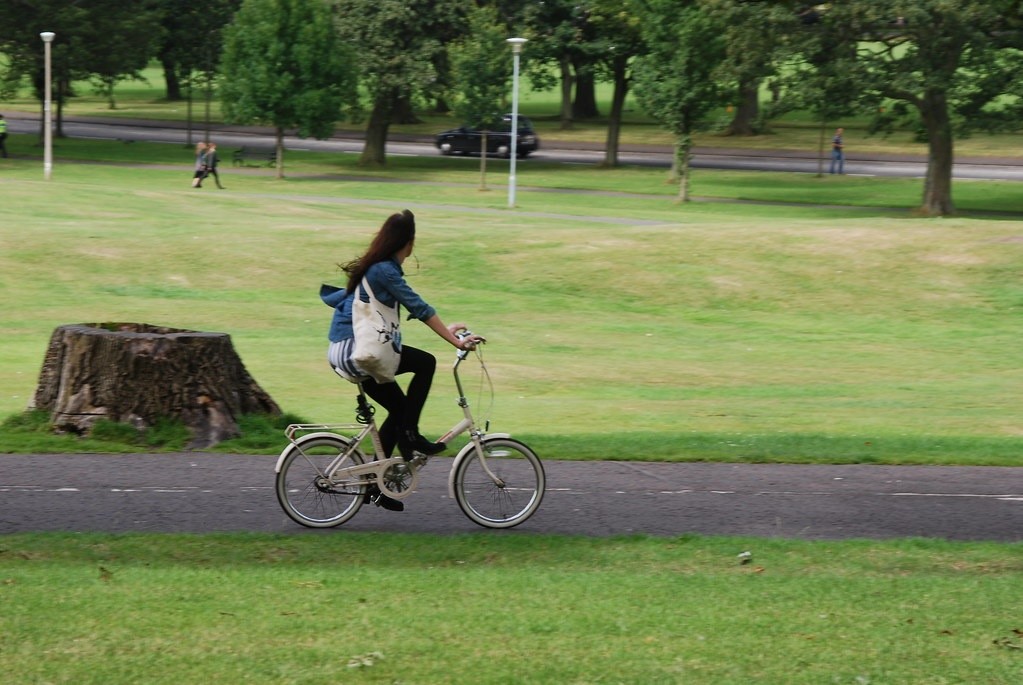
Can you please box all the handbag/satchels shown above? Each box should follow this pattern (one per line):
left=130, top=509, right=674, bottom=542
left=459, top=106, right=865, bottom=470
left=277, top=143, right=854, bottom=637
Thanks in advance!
left=351, top=275, right=402, bottom=384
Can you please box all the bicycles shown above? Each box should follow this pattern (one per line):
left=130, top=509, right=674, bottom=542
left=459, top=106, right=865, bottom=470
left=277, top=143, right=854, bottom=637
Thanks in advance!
left=273, top=328, right=547, bottom=530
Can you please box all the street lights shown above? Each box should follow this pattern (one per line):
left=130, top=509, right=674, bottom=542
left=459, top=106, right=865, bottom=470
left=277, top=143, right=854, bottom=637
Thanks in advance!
left=37, top=29, right=57, bottom=181
left=508, top=34, right=528, bottom=211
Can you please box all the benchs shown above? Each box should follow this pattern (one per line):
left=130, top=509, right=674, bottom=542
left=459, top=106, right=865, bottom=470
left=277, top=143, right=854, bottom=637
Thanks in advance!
left=233, top=147, right=277, bottom=168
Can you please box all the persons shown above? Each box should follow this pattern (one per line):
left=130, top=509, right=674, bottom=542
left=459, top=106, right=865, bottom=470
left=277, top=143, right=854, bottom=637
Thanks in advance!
left=195, top=142, right=226, bottom=189
left=828, top=127, right=845, bottom=175
left=0, top=114, right=9, bottom=158
left=191, top=142, right=209, bottom=187
left=316, top=209, right=487, bottom=513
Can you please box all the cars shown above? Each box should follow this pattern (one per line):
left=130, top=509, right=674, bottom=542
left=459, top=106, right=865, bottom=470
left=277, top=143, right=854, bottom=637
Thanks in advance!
left=436, top=115, right=541, bottom=159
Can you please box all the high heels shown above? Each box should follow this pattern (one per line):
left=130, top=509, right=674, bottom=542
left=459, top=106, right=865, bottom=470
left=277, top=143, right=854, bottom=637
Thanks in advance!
left=363, top=481, right=405, bottom=511
left=399, top=435, right=446, bottom=461
